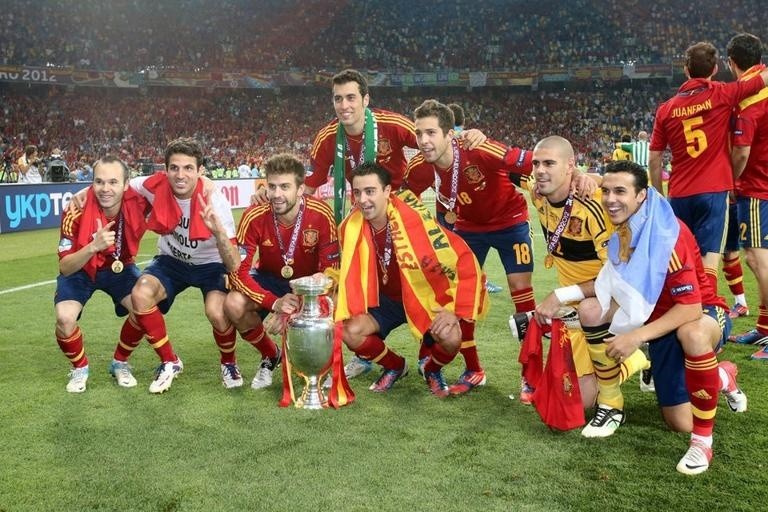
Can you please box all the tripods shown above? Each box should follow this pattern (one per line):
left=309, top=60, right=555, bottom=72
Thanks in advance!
left=0, top=164, right=16, bottom=183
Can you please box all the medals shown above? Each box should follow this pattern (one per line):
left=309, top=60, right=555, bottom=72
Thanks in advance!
left=281, top=265, right=294, bottom=279
left=111, top=260, right=124, bottom=273
left=444, top=212, right=457, bottom=225
left=544, top=255, right=554, bottom=269
left=383, top=273, right=388, bottom=285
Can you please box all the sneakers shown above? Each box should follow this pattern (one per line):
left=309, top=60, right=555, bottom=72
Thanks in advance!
left=718, top=366, right=747, bottom=413
left=675, top=433, right=714, bottom=477
left=65, top=365, right=90, bottom=394
left=368, top=356, right=408, bottom=393
left=250, top=343, right=282, bottom=390
left=341, top=354, right=371, bottom=382
left=218, top=361, right=244, bottom=390
left=417, top=356, right=486, bottom=400
left=728, top=303, right=749, bottom=319
left=520, top=370, right=535, bottom=406
left=727, top=329, right=768, bottom=361
left=640, top=367, right=656, bottom=393
left=149, top=355, right=184, bottom=395
left=109, top=359, right=137, bottom=388
left=581, top=403, right=625, bottom=438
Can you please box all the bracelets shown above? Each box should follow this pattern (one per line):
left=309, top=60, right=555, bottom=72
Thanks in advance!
left=554, top=284, right=585, bottom=304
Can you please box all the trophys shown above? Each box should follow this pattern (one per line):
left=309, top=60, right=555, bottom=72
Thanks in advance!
left=284, top=276, right=337, bottom=411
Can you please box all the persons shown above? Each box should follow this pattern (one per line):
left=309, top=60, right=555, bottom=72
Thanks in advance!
left=532, top=160, right=747, bottom=475
left=720, top=196, right=750, bottom=319
left=508, top=134, right=656, bottom=439
left=250, top=70, right=486, bottom=380
left=649, top=42, right=768, bottom=297
left=71, top=137, right=244, bottom=394
left=53, top=155, right=217, bottom=394
left=333, top=162, right=490, bottom=399
left=725, top=33, right=768, bottom=361
left=447, top=104, right=503, bottom=294
left=223, top=153, right=341, bottom=391
left=394, top=98, right=598, bottom=406
